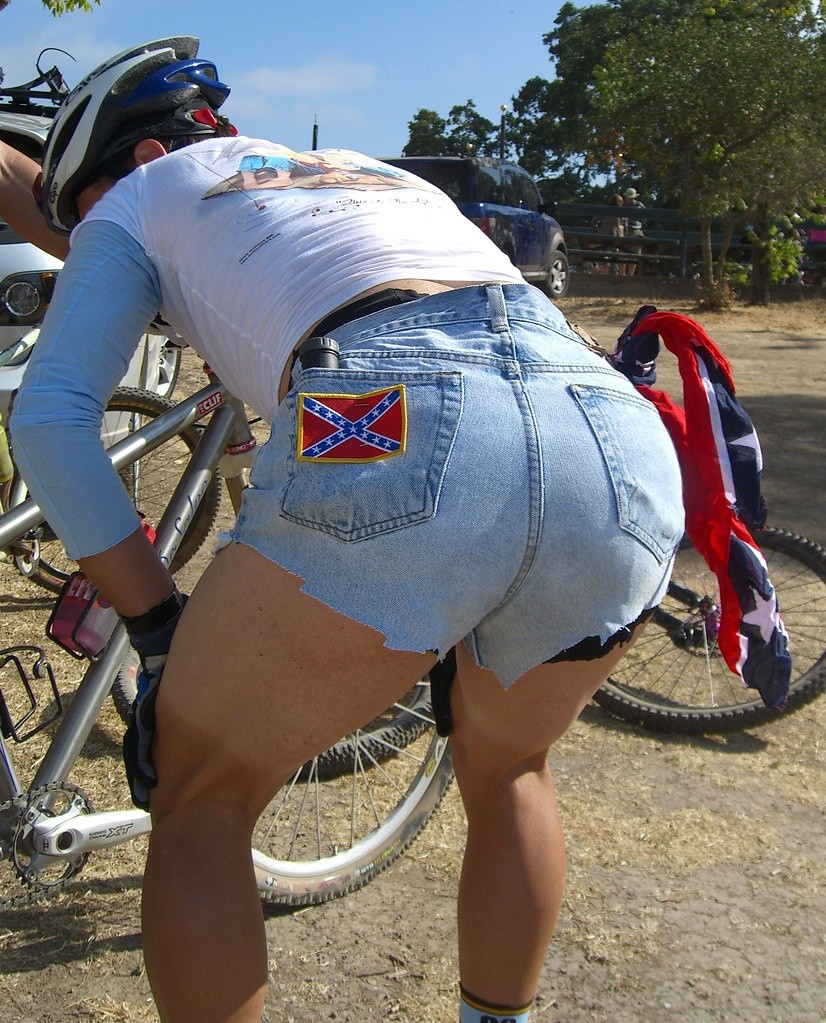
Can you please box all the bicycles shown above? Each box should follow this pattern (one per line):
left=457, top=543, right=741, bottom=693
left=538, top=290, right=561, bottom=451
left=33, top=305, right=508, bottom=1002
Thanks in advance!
left=0, top=330, right=825, bottom=913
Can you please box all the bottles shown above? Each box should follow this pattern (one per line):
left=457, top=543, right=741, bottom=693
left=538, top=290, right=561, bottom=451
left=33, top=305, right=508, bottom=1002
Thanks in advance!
left=53, top=522, right=156, bottom=654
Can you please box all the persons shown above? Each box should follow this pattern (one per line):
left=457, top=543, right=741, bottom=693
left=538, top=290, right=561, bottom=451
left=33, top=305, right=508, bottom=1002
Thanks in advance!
left=592, top=192, right=624, bottom=271
left=620, top=188, right=647, bottom=275
left=1, top=36, right=687, bottom=1023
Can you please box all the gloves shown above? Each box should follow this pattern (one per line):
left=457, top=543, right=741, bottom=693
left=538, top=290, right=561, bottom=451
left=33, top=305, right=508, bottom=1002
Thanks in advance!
left=117, top=582, right=192, bottom=812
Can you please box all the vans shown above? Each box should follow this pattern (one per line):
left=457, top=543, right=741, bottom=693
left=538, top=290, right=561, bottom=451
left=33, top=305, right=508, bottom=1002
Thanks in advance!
left=371, top=153, right=570, bottom=299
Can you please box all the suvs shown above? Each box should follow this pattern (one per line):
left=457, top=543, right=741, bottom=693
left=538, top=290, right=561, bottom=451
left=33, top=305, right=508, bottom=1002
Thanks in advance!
left=0, top=111, right=185, bottom=528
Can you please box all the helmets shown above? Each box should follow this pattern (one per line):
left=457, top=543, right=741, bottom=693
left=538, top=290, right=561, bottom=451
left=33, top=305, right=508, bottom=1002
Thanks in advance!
left=33, top=36, right=237, bottom=236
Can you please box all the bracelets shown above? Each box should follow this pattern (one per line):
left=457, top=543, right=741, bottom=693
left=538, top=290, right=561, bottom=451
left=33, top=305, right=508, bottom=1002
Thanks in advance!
left=117, top=586, right=183, bottom=634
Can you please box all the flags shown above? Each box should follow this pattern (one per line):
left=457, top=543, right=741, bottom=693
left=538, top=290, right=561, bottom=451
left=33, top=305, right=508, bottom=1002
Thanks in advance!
left=631, top=311, right=794, bottom=712
